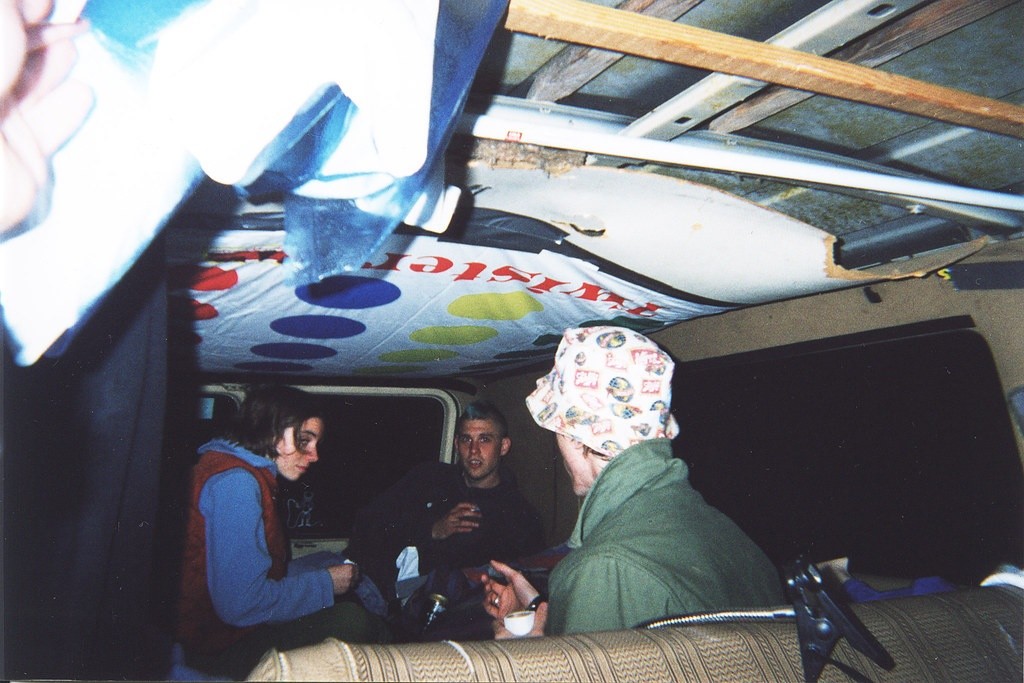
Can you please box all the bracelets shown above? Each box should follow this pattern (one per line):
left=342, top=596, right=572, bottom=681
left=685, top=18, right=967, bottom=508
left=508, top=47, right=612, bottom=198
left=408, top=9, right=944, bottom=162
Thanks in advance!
left=526, top=595, right=548, bottom=612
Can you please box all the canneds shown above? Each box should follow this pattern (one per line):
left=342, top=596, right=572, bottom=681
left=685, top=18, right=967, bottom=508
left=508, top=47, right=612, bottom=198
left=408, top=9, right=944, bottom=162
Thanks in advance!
left=416, top=594, right=449, bottom=635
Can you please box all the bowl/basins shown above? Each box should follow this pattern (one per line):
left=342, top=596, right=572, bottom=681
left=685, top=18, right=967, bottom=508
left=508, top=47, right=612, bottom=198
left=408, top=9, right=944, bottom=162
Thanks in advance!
left=504, top=611, right=535, bottom=636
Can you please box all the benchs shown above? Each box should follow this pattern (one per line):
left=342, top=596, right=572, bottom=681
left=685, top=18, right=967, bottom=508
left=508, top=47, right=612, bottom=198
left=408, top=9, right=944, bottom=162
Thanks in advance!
left=240, top=583, right=1023, bottom=683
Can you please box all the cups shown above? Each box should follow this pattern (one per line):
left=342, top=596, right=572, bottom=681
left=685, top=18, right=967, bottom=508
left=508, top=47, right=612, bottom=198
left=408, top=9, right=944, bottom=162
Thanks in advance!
left=460, top=504, right=483, bottom=522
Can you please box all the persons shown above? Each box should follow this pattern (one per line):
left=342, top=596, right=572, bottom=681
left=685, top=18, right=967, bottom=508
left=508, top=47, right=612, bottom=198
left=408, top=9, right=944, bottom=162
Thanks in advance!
left=171, top=381, right=391, bottom=683
left=340, top=396, right=544, bottom=643
left=482, top=326, right=787, bottom=642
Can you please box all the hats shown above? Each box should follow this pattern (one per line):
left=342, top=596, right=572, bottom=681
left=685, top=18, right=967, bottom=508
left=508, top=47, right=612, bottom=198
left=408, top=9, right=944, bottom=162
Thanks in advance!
left=525, top=325, right=681, bottom=458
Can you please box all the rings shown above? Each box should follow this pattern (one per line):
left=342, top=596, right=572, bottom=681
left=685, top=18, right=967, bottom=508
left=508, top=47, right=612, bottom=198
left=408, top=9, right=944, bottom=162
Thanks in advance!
left=491, top=597, right=499, bottom=606
